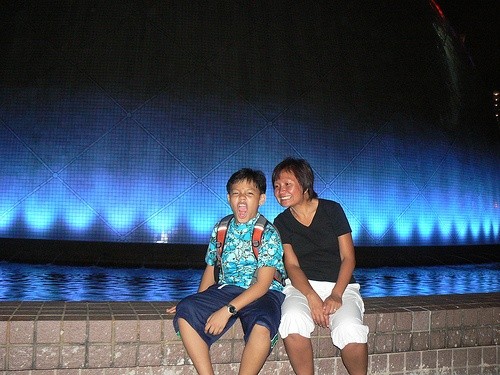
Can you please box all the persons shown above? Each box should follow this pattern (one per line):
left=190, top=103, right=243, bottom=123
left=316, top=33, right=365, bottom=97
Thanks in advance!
left=166, top=167, right=287, bottom=375
left=273, top=157, right=369, bottom=375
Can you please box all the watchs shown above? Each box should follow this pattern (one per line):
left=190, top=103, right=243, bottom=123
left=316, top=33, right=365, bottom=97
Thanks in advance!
left=225, top=302, right=239, bottom=316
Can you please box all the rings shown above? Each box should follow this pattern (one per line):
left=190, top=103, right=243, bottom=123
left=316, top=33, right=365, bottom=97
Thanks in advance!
left=333, top=309, right=337, bottom=312
